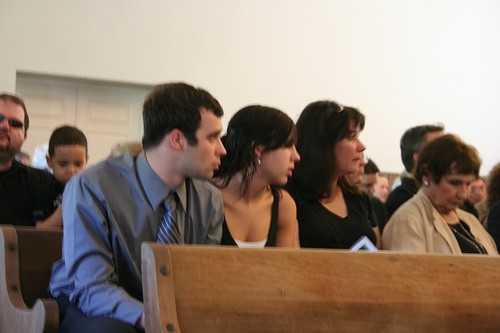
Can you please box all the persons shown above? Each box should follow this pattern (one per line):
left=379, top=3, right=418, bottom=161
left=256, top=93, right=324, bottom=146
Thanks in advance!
left=49, top=82, right=226, bottom=333
left=382, top=134, right=499, bottom=256
left=382, top=122, right=479, bottom=232
left=460, top=163, right=500, bottom=253
left=212, top=104, right=300, bottom=247
left=0, top=92, right=55, bottom=227
left=283, top=100, right=382, bottom=251
left=32, top=123, right=91, bottom=230
left=346, top=148, right=389, bottom=235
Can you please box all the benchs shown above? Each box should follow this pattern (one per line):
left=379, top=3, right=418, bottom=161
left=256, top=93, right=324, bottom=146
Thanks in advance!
left=0, top=224, right=66, bottom=333
left=138, top=241, right=500, bottom=333
